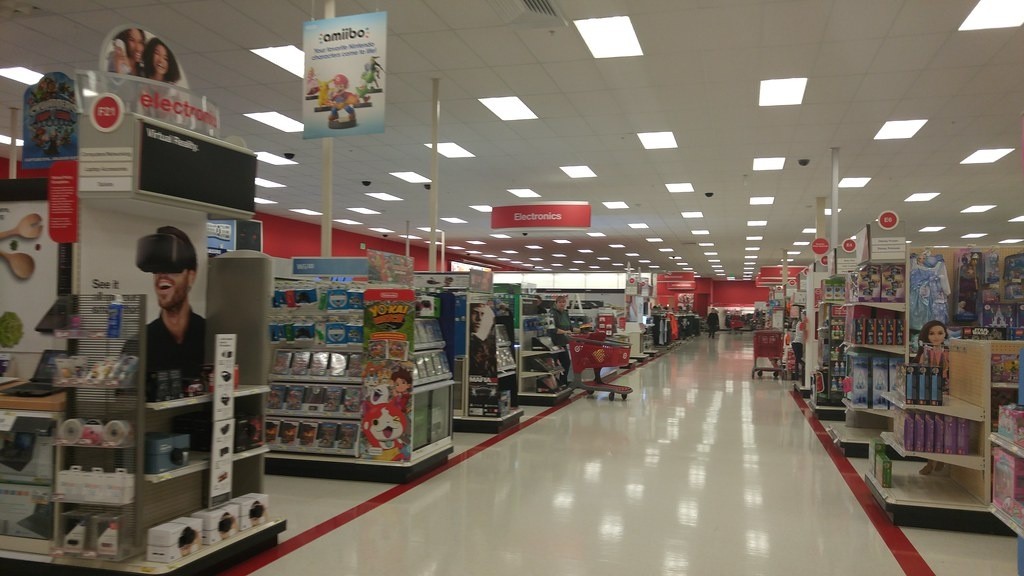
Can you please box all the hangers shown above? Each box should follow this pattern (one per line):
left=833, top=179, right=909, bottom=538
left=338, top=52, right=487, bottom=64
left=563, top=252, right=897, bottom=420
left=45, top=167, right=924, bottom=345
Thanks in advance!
left=922, top=247, right=932, bottom=256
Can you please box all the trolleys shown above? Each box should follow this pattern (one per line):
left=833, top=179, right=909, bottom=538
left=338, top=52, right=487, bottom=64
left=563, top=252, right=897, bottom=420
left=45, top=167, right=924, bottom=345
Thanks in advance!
left=752, top=326, right=787, bottom=379
left=566, top=331, right=633, bottom=401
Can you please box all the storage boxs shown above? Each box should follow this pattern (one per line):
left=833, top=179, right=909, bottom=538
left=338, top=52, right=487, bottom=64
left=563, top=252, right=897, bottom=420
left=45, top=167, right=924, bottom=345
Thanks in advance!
left=470, top=389, right=510, bottom=417
left=0, top=409, right=134, bottom=540
left=146, top=493, right=269, bottom=563
left=806, top=247, right=1024, bottom=526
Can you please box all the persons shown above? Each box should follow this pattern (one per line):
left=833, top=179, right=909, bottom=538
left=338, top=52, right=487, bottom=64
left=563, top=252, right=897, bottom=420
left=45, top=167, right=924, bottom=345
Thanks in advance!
left=534, top=296, right=548, bottom=315
left=792, top=318, right=807, bottom=381
left=175, top=527, right=202, bottom=557
left=113, top=226, right=207, bottom=403
left=915, top=320, right=949, bottom=365
left=217, top=512, right=240, bottom=541
left=705, top=307, right=720, bottom=339
left=626, top=296, right=637, bottom=321
left=468, top=303, right=497, bottom=379
left=106, top=28, right=182, bottom=86
left=548, top=296, right=582, bottom=390
left=248, top=501, right=271, bottom=526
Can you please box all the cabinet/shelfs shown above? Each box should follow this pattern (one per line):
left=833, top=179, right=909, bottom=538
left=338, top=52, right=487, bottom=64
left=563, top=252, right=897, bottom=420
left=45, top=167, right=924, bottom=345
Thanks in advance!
left=434, top=285, right=581, bottom=432
left=865, top=338, right=1016, bottom=538
left=767, top=306, right=813, bottom=397
left=987, top=431, right=1023, bottom=538
left=809, top=300, right=849, bottom=421
left=266, top=250, right=456, bottom=484
left=0, top=71, right=289, bottom=576
left=828, top=241, right=1024, bottom=459
